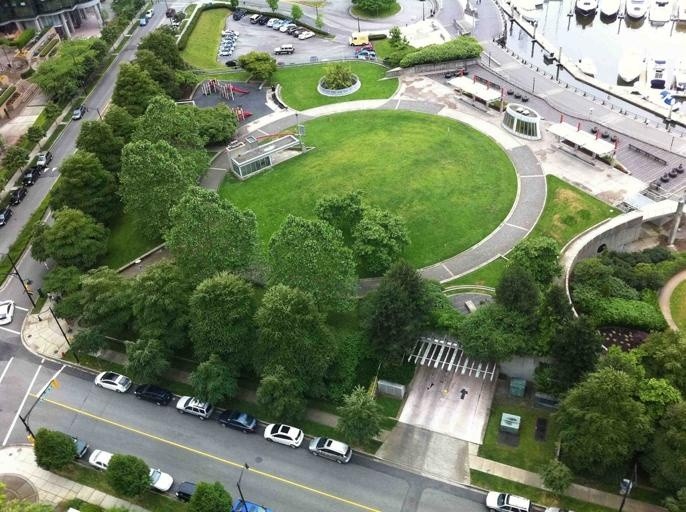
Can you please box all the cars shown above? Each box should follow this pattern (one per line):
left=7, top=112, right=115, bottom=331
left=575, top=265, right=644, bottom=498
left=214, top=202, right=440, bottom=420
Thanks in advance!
left=134, top=383, right=171, bottom=406
left=355, top=43, right=376, bottom=60
left=263, top=423, right=305, bottom=448
left=94, top=371, right=131, bottom=393
left=148, top=468, right=172, bottom=493
left=0, top=299, right=15, bottom=325
left=218, top=29, right=239, bottom=56
left=145, top=9, right=154, bottom=19
left=231, top=499, right=271, bottom=512
left=249, top=13, right=315, bottom=40
left=0, top=151, right=52, bottom=225
left=308, top=436, right=353, bottom=464
left=233, top=8, right=248, bottom=20
left=87, top=448, right=114, bottom=471
left=177, top=482, right=195, bottom=501
left=218, top=409, right=257, bottom=433
left=71, top=105, right=85, bottom=120
left=485, top=490, right=532, bottom=512
left=71, top=436, right=89, bottom=460
left=175, top=396, right=213, bottom=420
left=139, top=17, right=147, bottom=26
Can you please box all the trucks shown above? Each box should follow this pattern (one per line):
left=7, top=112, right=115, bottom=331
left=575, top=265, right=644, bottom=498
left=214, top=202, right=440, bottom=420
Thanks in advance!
left=349, top=34, right=369, bottom=46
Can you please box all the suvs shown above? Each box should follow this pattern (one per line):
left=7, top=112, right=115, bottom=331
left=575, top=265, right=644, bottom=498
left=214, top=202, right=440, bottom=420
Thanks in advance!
left=274, top=44, right=294, bottom=55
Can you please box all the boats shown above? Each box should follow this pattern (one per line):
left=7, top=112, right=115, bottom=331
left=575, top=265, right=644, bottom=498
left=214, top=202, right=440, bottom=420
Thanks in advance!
left=618, top=58, right=686, bottom=112
left=599, top=1, right=622, bottom=19
left=576, top=0, right=598, bottom=18
left=626, top=1, right=649, bottom=20
left=650, top=1, right=672, bottom=22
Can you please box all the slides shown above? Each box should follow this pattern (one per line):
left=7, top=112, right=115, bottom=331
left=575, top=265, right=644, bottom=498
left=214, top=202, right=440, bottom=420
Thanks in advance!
left=232, top=86, right=250, bottom=94
left=241, top=110, right=253, bottom=116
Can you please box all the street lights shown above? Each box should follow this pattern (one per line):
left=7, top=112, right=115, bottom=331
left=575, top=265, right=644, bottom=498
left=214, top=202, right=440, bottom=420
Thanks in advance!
left=236, top=461, right=249, bottom=512
left=618, top=461, right=638, bottom=512
left=37, top=307, right=80, bottom=364
